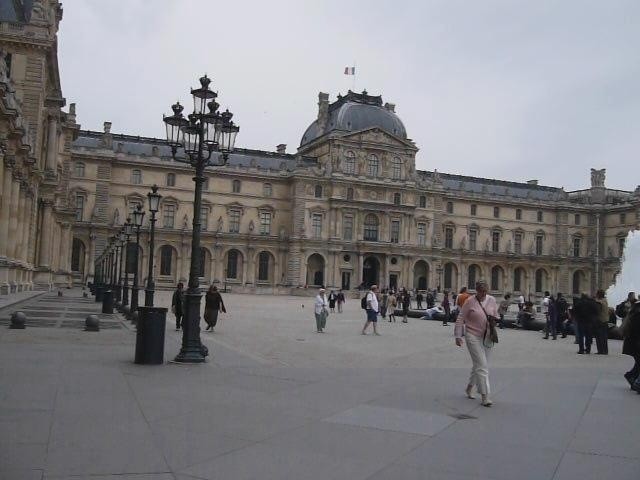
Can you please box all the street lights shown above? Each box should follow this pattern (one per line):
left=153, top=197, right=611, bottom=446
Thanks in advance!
left=123, top=217, right=134, bottom=307
left=94, top=228, right=125, bottom=302
left=131, top=201, right=145, bottom=311
left=144, top=183, right=162, bottom=306
left=161, top=72, right=235, bottom=362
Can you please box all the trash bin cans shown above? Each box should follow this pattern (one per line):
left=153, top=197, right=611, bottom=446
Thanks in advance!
left=95, top=287, right=104, bottom=302
left=134, top=306, right=168, bottom=365
left=102, top=290, right=113, bottom=314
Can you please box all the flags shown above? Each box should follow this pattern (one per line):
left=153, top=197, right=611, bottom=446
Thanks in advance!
left=344, top=66, right=355, bottom=75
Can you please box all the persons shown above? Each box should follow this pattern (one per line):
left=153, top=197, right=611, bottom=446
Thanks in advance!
left=376, top=286, right=423, bottom=323
left=360, top=285, right=380, bottom=336
left=608, top=292, right=640, bottom=325
left=452, top=279, right=501, bottom=407
left=542, top=291, right=568, bottom=340
left=621, top=306, right=639, bottom=394
left=315, top=287, right=346, bottom=333
left=170, top=283, right=186, bottom=331
left=570, top=289, right=613, bottom=356
left=424, top=287, right=471, bottom=336
left=203, top=285, right=227, bottom=332
left=497, top=292, right=535, bottom=329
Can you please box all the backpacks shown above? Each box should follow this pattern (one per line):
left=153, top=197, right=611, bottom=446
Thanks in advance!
left=361, top=292, right=373, bottom=309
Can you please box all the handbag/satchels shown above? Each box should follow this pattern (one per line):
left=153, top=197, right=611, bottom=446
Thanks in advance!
left=392, top=299, right=397, bottom=305
left=615, top=301, right=628, bottom=317
left=487, top=317, right=499, bottom=343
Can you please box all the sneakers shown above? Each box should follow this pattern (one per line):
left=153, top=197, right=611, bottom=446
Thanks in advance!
left=625, top=373, right=639, bottom=389
left=466, top=389, right=476, bottom=399
left=482, top=398, right=491, bottom=405
